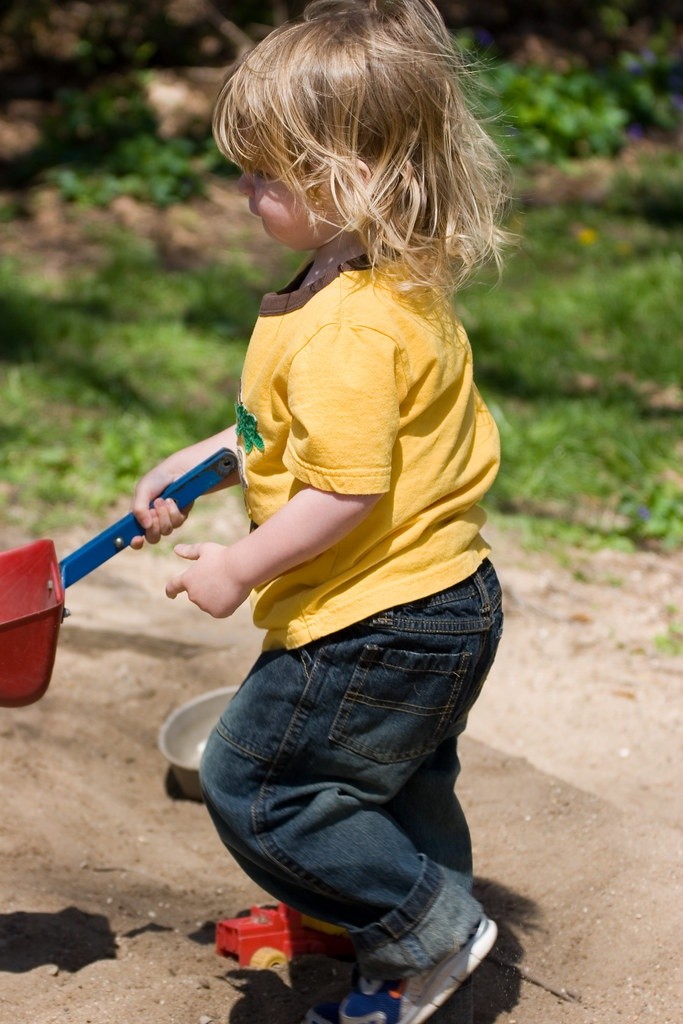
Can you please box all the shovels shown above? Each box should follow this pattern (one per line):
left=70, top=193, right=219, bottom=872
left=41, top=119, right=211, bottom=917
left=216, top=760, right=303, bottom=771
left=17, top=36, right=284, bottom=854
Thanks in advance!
left=1, top=449, right=239, bottom=710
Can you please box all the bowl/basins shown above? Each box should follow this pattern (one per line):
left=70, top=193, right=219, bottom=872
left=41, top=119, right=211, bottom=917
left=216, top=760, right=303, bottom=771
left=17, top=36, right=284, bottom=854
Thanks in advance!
left=158, top=684, right=243, bottom=798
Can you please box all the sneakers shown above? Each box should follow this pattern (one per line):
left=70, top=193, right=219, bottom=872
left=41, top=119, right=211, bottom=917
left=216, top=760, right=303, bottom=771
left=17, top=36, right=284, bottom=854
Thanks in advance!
left=306, top=914, right=498, bottom=1024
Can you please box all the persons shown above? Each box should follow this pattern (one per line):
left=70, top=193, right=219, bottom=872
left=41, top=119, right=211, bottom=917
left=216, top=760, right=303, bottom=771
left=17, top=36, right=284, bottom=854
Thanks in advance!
left=129, top=0, right=513, bottom=1024
